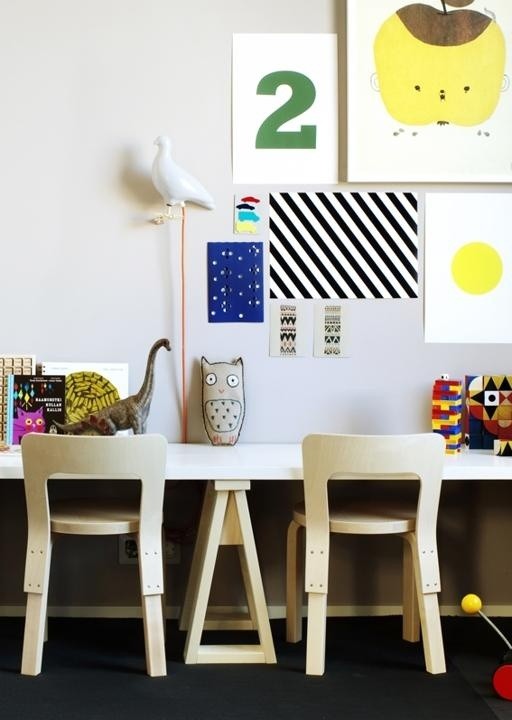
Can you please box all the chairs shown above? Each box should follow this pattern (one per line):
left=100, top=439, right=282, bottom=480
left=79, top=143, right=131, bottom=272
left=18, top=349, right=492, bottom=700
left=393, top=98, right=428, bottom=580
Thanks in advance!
left=285, top=431, right=446, bottom=677
left=19, top=432, right=169, bottom=678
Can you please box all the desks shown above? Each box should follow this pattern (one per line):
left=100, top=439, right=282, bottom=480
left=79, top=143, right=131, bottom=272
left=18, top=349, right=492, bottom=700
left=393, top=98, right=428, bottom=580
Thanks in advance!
left=0, top=431, right=512, bottom=618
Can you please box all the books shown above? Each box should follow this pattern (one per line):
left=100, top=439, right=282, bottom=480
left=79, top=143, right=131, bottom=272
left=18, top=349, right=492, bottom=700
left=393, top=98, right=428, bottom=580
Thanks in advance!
left=5, top=363, right=130, bottom=445
